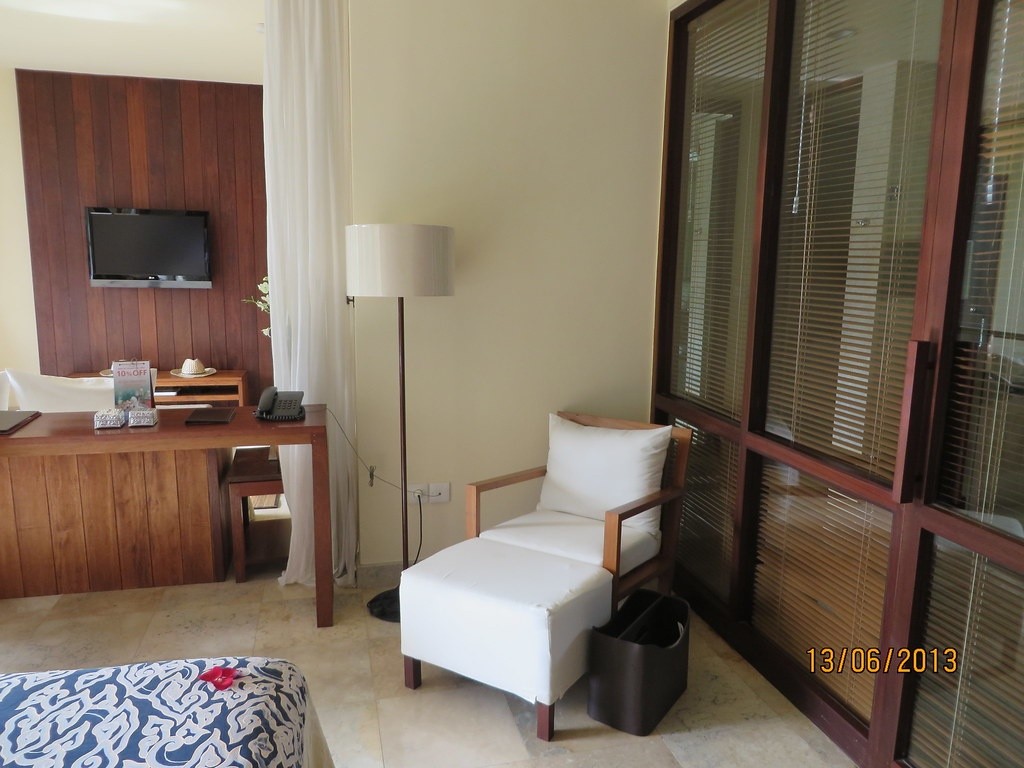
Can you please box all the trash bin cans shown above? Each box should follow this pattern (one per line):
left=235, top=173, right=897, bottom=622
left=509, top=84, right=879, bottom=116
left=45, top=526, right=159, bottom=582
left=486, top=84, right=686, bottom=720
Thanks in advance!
left=587, top=590, right=691, bottom=737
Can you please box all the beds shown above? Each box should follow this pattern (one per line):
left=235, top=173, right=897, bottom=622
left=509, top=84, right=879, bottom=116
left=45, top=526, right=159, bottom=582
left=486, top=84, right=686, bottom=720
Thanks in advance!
left=0, top=658, right=336, bottom=768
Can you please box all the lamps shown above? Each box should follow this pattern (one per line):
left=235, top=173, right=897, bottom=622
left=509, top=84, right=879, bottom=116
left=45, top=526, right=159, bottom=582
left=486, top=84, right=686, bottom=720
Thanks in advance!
left=346, top=224, right=455, bottom=620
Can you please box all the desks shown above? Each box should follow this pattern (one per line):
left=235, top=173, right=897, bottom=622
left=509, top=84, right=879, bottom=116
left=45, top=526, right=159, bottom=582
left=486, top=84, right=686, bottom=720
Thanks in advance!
left=1, top=402, right=334, bottom=628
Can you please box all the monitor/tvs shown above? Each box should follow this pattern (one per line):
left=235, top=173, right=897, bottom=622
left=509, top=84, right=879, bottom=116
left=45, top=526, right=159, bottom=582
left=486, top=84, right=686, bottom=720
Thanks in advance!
left=85, top=206, right=212, bottom=290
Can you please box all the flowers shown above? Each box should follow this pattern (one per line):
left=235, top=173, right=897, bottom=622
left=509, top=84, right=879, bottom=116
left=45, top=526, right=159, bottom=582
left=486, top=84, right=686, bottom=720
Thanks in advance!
left=240, top=273, right=271, bottom=338
left=199, top=666, right=235, bottom=690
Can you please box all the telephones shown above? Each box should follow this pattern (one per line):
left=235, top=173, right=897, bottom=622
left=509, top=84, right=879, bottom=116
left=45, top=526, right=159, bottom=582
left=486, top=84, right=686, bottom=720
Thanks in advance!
left=254, top=386, right=305, bottom=421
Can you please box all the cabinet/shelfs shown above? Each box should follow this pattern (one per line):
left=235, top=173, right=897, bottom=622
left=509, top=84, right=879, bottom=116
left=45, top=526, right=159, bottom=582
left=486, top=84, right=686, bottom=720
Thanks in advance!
left=64, top=368, right=251, bottom=405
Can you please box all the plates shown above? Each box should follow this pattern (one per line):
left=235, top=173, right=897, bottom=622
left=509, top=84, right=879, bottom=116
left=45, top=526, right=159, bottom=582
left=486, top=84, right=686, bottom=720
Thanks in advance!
left=99, top=370, right=113, bottom=376
left=170, top=368, right=216, bottom=379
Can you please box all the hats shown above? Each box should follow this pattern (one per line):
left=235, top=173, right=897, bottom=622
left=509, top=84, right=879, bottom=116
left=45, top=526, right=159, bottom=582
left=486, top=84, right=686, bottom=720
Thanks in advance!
left=99, top=359, right=129, bottom=376
left=169, top=358, right=217, bottom=378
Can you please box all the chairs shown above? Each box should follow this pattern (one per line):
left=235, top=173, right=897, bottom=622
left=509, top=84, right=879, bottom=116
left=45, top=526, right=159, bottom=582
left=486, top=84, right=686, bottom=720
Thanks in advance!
left=228, top=447, right=286, bottom=584
left=402, top=413, right=698, bottom=740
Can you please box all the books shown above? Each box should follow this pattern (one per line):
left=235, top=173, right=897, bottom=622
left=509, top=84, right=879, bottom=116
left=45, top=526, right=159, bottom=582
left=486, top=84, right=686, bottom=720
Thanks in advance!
left=184, top=407, right=236, bottom=426
left=0, top=411, right=42, bottom=436
